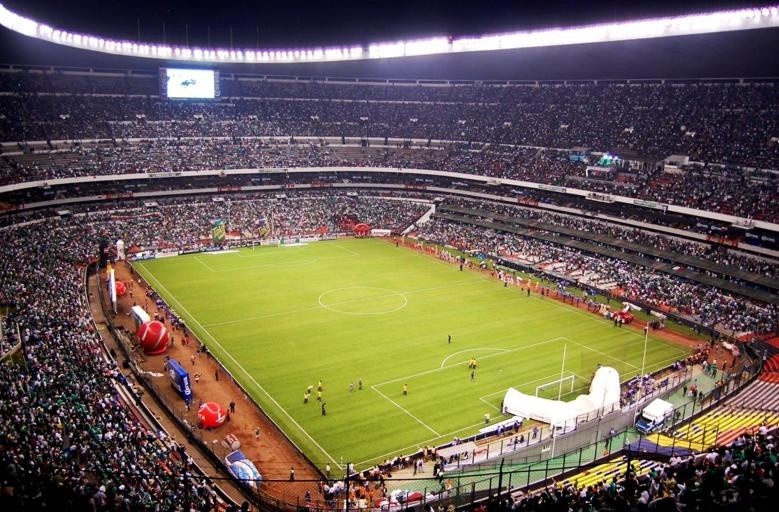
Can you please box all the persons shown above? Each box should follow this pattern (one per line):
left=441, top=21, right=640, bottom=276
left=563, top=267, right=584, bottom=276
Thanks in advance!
left=1, top=69, right=777, bottom=509
